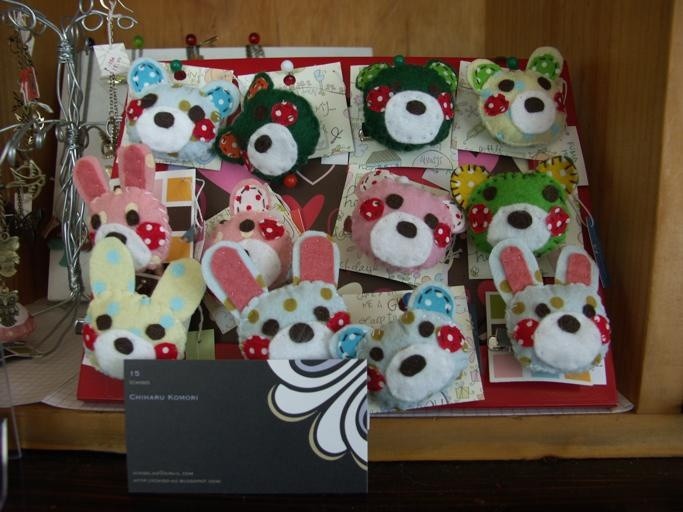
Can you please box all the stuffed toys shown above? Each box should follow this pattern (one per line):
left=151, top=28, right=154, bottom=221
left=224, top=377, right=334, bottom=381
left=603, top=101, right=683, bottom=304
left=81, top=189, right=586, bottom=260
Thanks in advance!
left=74, top=48, right=610, bottom=404
left=73, top=46, right=612, bottom=405
